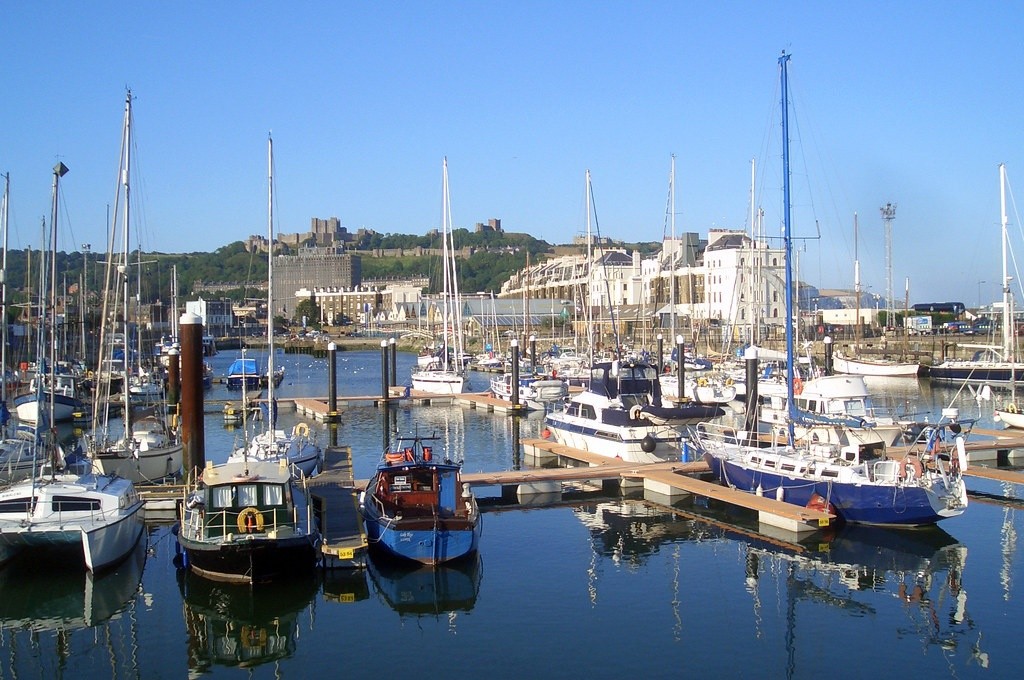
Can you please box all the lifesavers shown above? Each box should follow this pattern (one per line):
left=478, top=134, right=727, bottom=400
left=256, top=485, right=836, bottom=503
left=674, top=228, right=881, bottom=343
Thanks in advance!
left=931, top=438, right=941, bottom=461
left=237, top=508, right=264, bottom=533
left=899, top=457, right=923, bottom=478
left=725, top=378, right=734, bottom=386
left=1008, top=403, right=1017, bottom=414
left=793, top=378, right=802, bottom=395
left=698, top=379, right=707, bottom=387
left=295, top=422, right=309, bottom=437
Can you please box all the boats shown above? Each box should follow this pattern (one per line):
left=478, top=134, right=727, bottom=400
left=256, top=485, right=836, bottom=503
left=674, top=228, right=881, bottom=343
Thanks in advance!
left=362, top=543, right=485, bottom=618
left=175, top=563, right=323, bottom=635
left=360, top=422, right=482, bottom=569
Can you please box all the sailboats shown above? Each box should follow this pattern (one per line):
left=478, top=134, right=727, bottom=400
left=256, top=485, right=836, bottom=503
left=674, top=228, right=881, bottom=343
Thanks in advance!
left=0, top=45, right=1024, bottom=581
left=0, top=527, right=152, bottom=677
